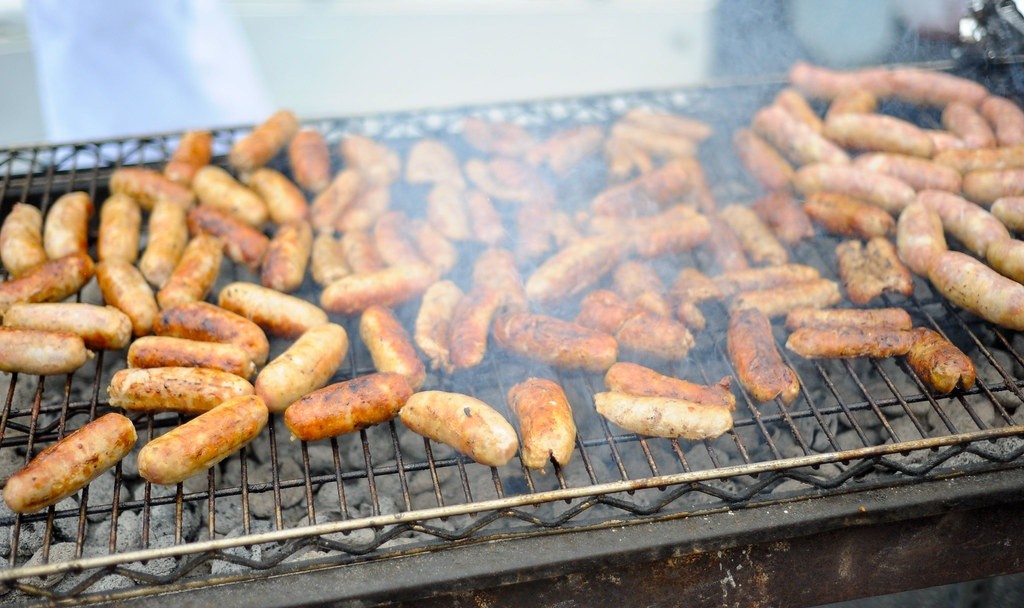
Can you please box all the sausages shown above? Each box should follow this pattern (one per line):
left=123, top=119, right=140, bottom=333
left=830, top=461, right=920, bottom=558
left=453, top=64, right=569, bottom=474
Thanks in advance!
left=2, top=61, right=1024, bottom=514
